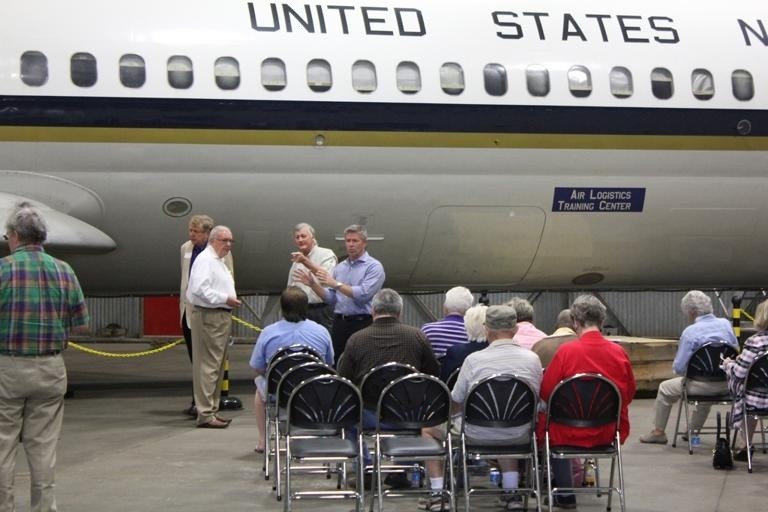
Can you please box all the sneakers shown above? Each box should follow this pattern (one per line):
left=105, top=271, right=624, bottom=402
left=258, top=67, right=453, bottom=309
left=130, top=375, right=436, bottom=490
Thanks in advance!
left=681, top=429, right=689, bottom=441
left=497, top=492, right=525, bottom=512
left=416, top=495, right=450, bottom=512
left=638, top=429, right=668, bottom=444
left=383, top=473, right=413, bottom=490
left=544, top=493, right=577, bottom=510
left=347, top=476, right=382, bottom=491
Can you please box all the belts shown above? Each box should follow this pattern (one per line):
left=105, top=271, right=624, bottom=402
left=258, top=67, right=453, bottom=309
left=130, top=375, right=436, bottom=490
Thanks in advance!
left=335, top=313, right=372, bottom=321
left=306, top=303, right=330, bottom=308
left=193, top=303, right=233, bottom=312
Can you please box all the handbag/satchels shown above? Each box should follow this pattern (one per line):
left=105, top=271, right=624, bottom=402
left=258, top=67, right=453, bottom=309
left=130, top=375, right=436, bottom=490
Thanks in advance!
left=710, top=438, right=734, bottom=470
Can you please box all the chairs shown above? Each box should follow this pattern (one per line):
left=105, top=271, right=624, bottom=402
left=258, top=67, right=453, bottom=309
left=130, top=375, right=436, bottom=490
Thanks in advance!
left=732, top=353, right=768, bottom=473
left=672, top=341, right=740, bottom=454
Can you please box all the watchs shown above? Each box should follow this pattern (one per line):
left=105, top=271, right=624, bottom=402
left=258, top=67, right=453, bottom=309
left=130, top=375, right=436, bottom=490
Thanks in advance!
left=334, top=281, right=342, bottom=290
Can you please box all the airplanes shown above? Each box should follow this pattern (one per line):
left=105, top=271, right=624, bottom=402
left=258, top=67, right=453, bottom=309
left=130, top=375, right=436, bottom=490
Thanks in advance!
left=0, top=0, right=767, bottom=300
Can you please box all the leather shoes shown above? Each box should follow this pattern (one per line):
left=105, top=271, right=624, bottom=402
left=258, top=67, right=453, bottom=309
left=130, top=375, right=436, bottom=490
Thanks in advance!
left=184, top=399, right=232, bottom=429
left=734, top=442, right=754, bottom=462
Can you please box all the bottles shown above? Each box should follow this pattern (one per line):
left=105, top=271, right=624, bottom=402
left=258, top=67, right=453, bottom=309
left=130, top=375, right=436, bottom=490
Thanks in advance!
left=584, top=459, right=595, bottom=486
left=412, top=462, right=420, bottom=488
left=691, top=428, right=701, bottom=450
left=490, top=467, right=501, bottom=487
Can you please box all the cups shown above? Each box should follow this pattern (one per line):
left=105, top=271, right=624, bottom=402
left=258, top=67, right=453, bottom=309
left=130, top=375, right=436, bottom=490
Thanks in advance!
left=290, top=252, right=302, bottom=260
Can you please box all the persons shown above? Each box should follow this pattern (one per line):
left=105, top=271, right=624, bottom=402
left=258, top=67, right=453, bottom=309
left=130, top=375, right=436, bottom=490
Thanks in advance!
left=184, top=225, right=246, bottom=428
left=716, top=296, right=768, bottom=463
left=415, top=286, right=636, bottom=512
left=283, top=219, right=337, bottom=337
left=333, top=286, right=447, bottom=488
left=247, top=285, right=338, bottom=458
left=638, top=288, right=741, bottom=445
left=179, top=214, right=236, bottom=417
left=290, top=224, right=389, bottom=368
left=0, top=209, right=91, bottom=510
